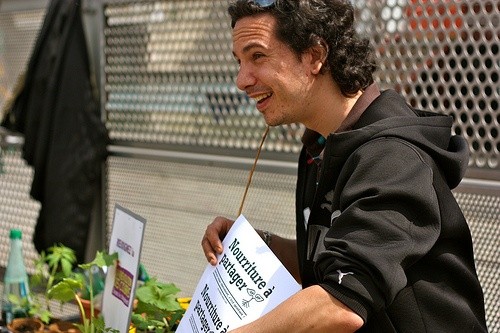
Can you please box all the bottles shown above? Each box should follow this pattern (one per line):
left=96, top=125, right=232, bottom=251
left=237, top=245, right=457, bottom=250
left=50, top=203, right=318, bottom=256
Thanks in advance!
left=5, top=229, right=33, bottom=327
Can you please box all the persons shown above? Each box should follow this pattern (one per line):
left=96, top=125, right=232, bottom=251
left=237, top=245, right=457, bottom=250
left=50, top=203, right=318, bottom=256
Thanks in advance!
left=202, top=1, right=488, bottom=333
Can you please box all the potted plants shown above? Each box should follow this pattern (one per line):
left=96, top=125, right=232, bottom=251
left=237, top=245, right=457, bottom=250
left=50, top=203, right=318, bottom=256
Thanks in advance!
left=6, top=239, right=186, bottom=332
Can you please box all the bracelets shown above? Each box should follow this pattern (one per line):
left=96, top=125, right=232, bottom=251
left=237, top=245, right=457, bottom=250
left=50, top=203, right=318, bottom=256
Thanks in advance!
left=263, top=231, right=272, bottom=245
